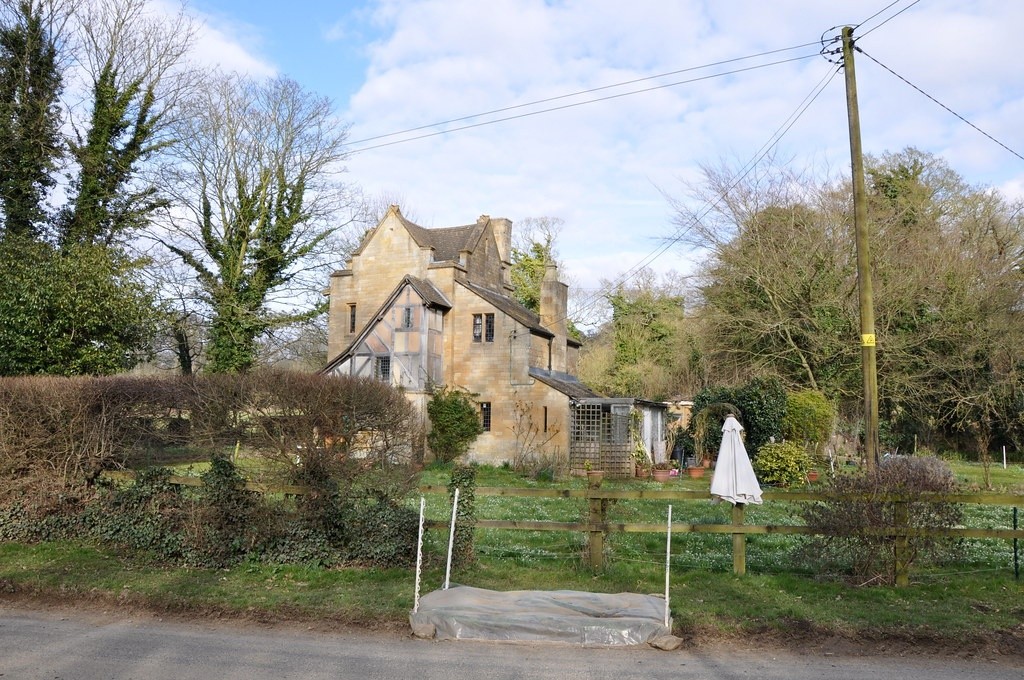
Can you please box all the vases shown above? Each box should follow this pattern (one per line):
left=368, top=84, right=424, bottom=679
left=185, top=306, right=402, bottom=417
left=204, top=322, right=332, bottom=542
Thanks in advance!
left=688, top=467, right=704, bottom=478
left=702, top=459, right=710, bottom=468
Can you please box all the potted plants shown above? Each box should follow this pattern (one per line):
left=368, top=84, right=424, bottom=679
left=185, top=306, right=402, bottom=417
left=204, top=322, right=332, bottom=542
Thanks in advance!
left=636, top=462, right=651, bottom=479
left=651, top=463, right=672, bottom=482
left=807, top=463, right=818, bottom=481
left=585, top=458, right=604, bottom=484
